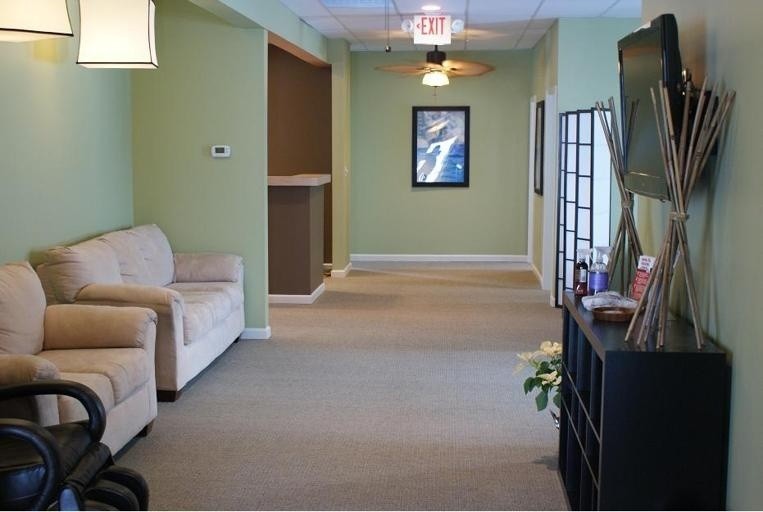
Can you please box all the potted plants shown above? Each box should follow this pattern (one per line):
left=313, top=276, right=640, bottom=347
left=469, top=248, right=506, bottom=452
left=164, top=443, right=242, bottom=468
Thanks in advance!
left=533, top=99, right=543, bottom=196
left=411, top=103, right=469, bottom=186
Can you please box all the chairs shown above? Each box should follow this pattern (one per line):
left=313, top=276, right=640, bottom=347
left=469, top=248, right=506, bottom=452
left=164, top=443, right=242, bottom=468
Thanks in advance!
left=2, top=377, right=155, bottom=510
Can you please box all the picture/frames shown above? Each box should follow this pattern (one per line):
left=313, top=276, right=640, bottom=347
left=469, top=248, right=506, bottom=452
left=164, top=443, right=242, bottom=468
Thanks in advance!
left=533, top=99, right=543, bottom=196
left=411, top=103, right=469, bottom=186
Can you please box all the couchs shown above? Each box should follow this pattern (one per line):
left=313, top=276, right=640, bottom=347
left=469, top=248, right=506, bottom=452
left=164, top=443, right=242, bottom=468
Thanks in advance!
left=36, top=223, right=245, bottom=402
left=3, top=260, right=158, bottom=459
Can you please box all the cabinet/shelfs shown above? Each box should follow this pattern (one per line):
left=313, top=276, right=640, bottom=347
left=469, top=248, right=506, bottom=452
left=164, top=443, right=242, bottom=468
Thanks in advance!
left=555, top=289, right=732, bottom=510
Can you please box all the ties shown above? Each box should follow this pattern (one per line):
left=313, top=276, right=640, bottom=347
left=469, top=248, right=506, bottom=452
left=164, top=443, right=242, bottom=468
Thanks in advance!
left=616, top=14, right=684, bottom=201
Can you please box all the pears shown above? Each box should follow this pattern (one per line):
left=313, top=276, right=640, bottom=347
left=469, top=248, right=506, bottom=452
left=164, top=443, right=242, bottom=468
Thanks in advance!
left=586, top=246, right=615, bottom=293
left=573, top=248, right=594, bottom=295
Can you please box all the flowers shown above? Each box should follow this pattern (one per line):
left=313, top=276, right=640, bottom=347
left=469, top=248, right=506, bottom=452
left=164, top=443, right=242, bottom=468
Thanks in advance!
left=514, top=340, right=565, bottom=428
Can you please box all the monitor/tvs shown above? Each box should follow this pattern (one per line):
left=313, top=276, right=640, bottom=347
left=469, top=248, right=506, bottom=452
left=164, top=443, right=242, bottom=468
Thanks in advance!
left=630, top=255, right=657, bottom=300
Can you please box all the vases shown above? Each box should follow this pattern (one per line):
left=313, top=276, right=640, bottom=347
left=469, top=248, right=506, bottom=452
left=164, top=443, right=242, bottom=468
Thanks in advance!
left=514, top=340, right=565, bottom=428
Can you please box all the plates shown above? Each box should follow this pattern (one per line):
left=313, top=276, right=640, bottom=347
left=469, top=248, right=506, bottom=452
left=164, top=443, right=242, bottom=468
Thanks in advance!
left=555, top=289, right=732, bottom=510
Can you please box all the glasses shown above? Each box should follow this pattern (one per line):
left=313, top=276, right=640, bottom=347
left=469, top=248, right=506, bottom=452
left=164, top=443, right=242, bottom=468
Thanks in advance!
left=371, top=2, right=497, bottom=87
left=2, top=1, right=161, bottom=69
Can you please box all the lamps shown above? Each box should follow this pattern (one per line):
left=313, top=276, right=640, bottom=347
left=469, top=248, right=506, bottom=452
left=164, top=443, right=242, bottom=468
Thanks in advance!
left=2, top=377, right=155, bottom=510
left=2, top=1, right=161, bottom=69
left=371, top=2, right=497, bottom=87
